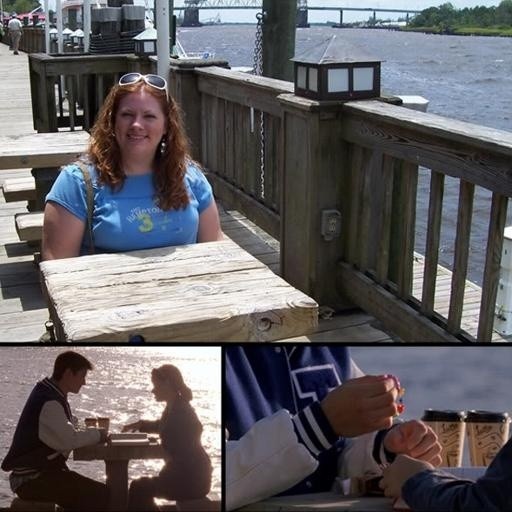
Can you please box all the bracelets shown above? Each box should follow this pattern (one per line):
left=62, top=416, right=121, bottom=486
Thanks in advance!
left=382, top=370, right=405, bottom=421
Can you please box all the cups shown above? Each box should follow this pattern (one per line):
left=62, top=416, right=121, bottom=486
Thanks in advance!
left=84, top=418, right=97, bottom=426
left=98, top=417, right=110, bottom=432
left=421, top=408, right=465, bottom=468
left=463, top=408, right=511, bottom=467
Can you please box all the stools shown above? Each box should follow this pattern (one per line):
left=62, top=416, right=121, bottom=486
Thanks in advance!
left=15, top=210, right=44, bottom=267
left=10, top=497, right=55, bottom=512
left=2, top=176, right=36, bottom=212
left=176, top=496, right=211, bottom=512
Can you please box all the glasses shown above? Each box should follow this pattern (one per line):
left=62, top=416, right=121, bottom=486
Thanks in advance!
left=118, top=73, right=169, bottom=102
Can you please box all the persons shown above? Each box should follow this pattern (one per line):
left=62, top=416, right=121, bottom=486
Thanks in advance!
left=38, top=72, right=220, bottom=341
left=225, top=347, right=448, bottom=509
left=8, top=13, right=24, bottom=57
left=120, top=362, right=215, bottom=511
left=376, top=432, right=511, bottom=512
left=1, top=350, right=108, bottom=512
